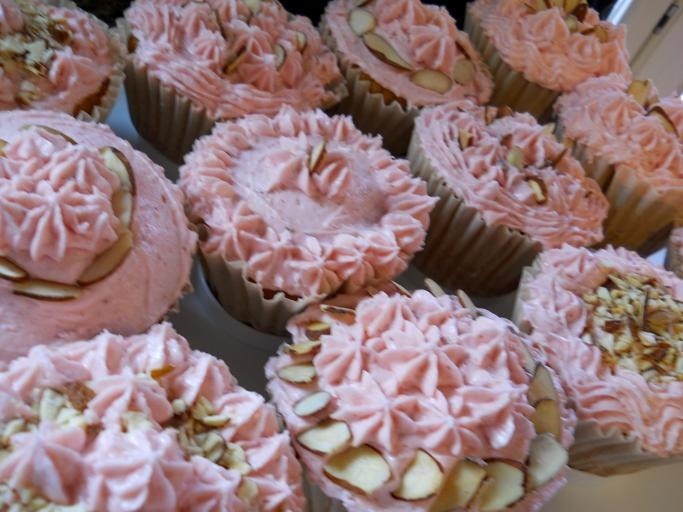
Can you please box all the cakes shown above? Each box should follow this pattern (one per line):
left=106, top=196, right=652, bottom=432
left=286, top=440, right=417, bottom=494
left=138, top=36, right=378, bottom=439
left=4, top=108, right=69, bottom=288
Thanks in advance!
left=512, top=242, right=683, bottom=478
left=112, top=0, right=351, bottom=164
left=175, top=102, right=442, bottom=339
left=462, top=1, right=634, bottom=123
left=0, top=320, right=310, bottom=512
left=0, top=108, right=200, bottom=373
left=551, top=73, right=683, bottom=260
left=668, top=226, right=683, bottom=279
left=265, top=277, right=578, bottom=511
left=316, top=1, right=496, bottom=158
left=0, top=0, right=127, bottom=123
left=402, top=99, right=611, bottom=298
